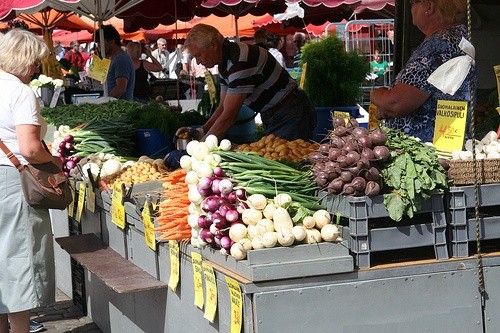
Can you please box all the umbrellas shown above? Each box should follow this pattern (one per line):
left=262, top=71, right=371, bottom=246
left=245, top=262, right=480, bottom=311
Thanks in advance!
left=0, top=0, right=90, bottom=74
left=122, top=0, right=288, bottom=41
left=41, top=0, right=149, bottom=97
left=273, top=0, right=402, bottom=27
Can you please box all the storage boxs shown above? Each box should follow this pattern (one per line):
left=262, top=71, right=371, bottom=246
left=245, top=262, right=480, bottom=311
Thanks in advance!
left=314, top=107, right=359, bottom=144
left=444, top=184, right=500, bottom=256
left=134, top=128, right=175, bottom=156
left=315, top=184, right=449, bottom=269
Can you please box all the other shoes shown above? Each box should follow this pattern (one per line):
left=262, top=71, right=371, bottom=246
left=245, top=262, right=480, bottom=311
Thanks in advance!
left=28, top=321, right=44, bottom=333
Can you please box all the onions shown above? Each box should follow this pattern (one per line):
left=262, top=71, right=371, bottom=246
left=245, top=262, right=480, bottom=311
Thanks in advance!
left=51, top=125, right=164, bottom=178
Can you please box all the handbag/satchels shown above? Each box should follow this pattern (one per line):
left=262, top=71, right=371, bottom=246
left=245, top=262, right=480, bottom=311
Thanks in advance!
left=18, top=160, right=73, bottom=210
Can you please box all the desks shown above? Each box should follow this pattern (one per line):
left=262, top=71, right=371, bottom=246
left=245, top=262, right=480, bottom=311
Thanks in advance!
left=49, top=183, right=500, bottom=333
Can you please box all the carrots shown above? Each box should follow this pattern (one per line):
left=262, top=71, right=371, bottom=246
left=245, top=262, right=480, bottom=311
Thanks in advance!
left=151, top=168, right=192, bottom=240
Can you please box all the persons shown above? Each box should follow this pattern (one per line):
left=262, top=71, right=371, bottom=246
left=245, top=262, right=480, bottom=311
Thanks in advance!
left=54, top=38, right=219, bottom=100
left=253, top=30, right=286, bottom=68
left=175, top=23, right=317, bottom=142
left=94, top=25, right=136, bottom=100
left=0, top=27, right=64, bottom=333
left=371, top=1, right=477, bottom=142
left=292, top=32, right=306, bottom=67
left=369, top=49, right=388, bottom=74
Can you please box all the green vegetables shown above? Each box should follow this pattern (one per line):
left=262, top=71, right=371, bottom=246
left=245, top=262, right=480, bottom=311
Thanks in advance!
left=117, top=100, right=209, bottom=128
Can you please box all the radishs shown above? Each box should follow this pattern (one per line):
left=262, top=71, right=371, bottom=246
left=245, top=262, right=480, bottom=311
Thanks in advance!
left=180, top=135, right=231, bottom=248
left=425, top=130, right=500, bottom=159
left=230, top=194, right=339, bottom=260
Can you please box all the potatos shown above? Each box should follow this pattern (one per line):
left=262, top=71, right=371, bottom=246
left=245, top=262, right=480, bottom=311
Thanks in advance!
left=110, top=161, right=168, bottom=185
left=237, top=134, right=319, bottom=162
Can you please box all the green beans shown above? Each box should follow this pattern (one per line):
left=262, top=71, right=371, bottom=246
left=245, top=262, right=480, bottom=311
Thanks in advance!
left=40, top=99, right=143, bottom=124
